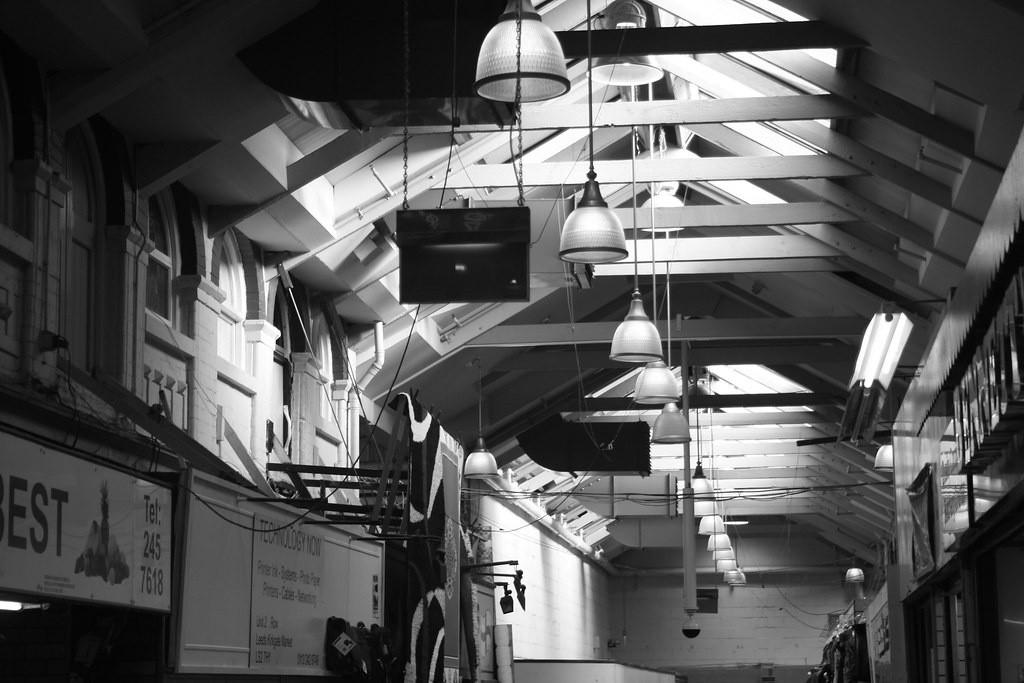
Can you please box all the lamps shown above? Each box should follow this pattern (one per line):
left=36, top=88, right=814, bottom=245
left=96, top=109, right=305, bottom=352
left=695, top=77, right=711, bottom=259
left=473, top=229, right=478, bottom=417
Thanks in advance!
left=462, top=0, right=948, bottom=613
